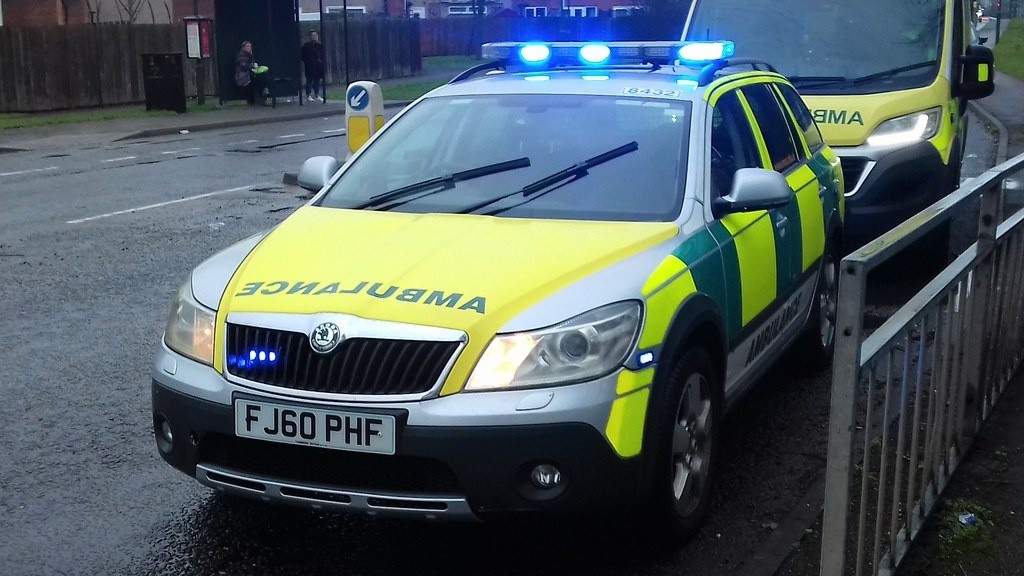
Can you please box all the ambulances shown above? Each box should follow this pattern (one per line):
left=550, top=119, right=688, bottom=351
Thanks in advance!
left=680, top=0, right=994, bottom=247
left=149, top=40, right=844, bottom=540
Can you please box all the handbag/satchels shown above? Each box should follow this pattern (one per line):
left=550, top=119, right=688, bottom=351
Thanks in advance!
left=251, top=65, right=269, bottom=74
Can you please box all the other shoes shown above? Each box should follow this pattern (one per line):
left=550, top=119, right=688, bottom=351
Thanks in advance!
left=265, top=87, right=269, bottom=94
left=306, top=96, right=313, bottom=102
left=264, top=97, right=271, bottom=106
left=316, top=96, right=324, bottom=101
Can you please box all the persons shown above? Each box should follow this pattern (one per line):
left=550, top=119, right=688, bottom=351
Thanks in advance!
left=301, top=30, right=326, bottom=102
left=235, top=41, right=273, bottom=106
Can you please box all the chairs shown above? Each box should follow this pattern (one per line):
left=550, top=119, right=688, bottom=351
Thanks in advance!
left=500, top=109, right=578, bottom=200
